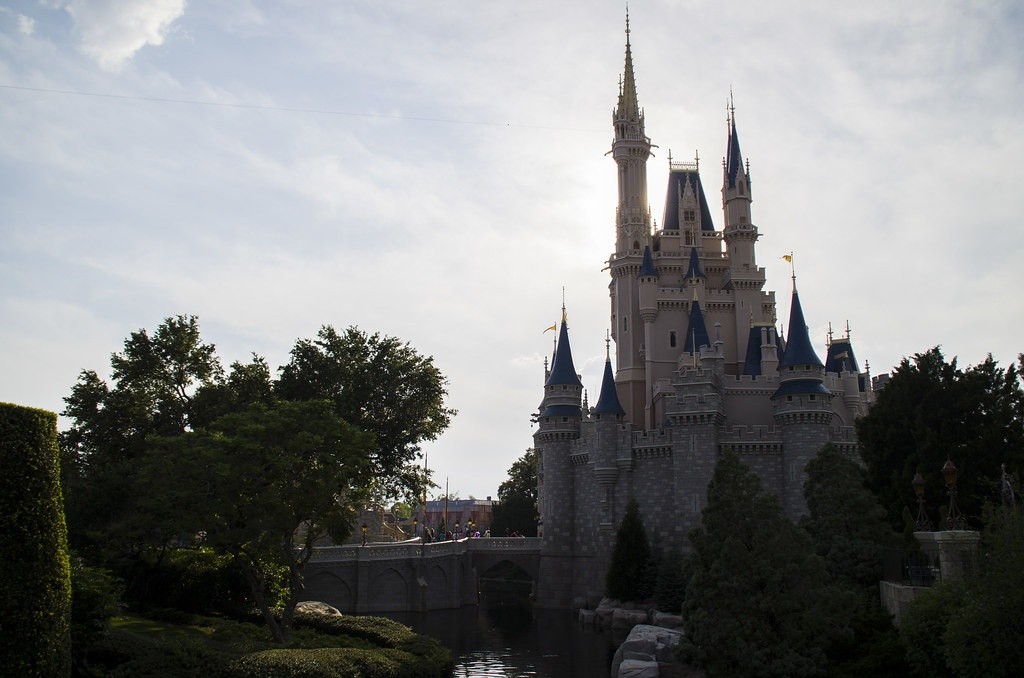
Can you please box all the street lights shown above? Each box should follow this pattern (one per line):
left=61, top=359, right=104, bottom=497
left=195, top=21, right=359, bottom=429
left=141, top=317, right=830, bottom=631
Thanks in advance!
left=472, top=521, right=476, bottom=535
left=414, top=517, right=418, bottom=538
left=394, top=507, right=400, bottom=531
left=362, top=523, right=367, bottom=545
left=468, top=518, right=472, bottom=537
left=455, top=522, right=459, bottom=540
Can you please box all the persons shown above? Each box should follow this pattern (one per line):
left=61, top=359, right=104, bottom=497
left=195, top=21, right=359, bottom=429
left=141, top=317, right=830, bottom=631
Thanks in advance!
left=470, top=528, right=527, bottom=545
left=424, top=526, right=453, bottom=543
